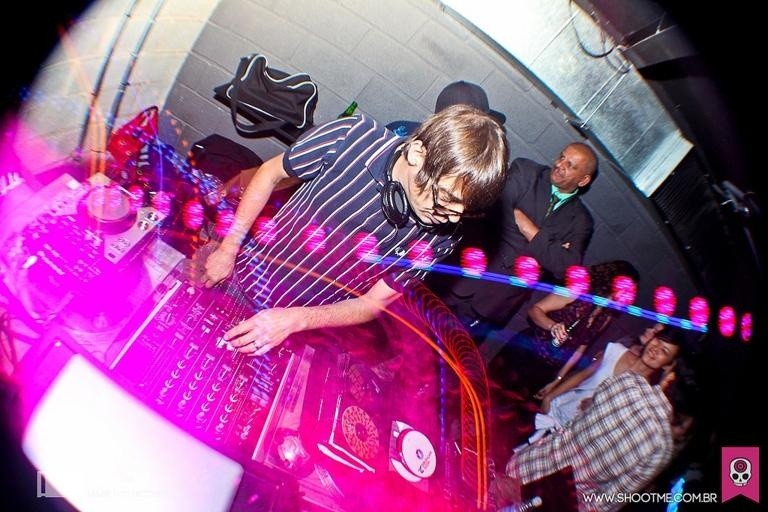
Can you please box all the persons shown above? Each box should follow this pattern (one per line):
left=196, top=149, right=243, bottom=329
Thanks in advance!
left=418, top=142, right=599, bottom=337
left=492, top=255, right=641, bottom=404
left=385, top=74, right=508, bottom=142
left=492, top=314, right=728, bottom=512
left=195, top=101, right=511, bottom=359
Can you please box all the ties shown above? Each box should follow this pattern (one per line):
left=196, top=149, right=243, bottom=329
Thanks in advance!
left=543, top=192, right=561, bottom=224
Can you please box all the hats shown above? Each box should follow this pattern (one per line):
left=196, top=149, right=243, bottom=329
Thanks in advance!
left=434, top=81, right=507, bottom=126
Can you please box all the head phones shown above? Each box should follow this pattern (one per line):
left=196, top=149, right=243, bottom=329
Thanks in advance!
left=381, top=155, right=439, bottom=231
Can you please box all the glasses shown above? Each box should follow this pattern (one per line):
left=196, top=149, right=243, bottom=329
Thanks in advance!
left=430, top=185, right=485, bottom=219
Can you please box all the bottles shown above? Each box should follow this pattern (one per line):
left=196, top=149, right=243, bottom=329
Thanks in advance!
left=336, top=102, right=359, bottom=118
left=551, top=314, right=583, bottom=348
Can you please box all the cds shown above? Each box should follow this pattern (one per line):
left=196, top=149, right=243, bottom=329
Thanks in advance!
left=401, top=431, right=438, bottom=479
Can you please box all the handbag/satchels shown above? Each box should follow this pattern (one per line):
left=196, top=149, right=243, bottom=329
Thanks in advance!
left=224, top=52, right=319, bottom=134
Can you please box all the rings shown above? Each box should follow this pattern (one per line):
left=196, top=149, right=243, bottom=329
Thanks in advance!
left=252, top=340, right=260, bottom=350
